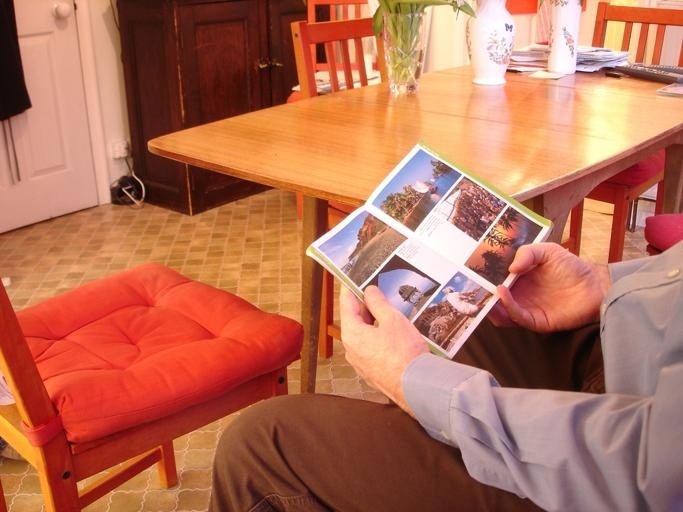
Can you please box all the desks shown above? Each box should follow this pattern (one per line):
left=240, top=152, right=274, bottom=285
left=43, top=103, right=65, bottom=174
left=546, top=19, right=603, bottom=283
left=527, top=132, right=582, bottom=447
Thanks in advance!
left=147, top=52, right=683, bottom=395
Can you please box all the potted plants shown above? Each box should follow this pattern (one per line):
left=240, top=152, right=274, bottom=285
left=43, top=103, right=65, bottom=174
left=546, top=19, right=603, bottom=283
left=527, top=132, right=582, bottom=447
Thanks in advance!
left=368, top=0, right=472, bottom=93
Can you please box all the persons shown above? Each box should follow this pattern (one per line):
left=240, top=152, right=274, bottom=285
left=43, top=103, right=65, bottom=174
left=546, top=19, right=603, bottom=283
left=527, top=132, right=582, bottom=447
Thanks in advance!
left=206, top=237, right=682, bottom=512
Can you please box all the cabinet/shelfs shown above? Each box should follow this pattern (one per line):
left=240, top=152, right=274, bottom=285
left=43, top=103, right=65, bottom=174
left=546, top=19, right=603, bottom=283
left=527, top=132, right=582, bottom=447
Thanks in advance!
left=116, top=1, right=330, bottom=217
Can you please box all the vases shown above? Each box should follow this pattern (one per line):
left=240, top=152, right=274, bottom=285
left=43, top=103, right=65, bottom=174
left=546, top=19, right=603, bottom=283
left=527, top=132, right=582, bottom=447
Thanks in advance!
left=536, top=1, right=582, bottom=76
left=464, top=0, right=516, bottom=86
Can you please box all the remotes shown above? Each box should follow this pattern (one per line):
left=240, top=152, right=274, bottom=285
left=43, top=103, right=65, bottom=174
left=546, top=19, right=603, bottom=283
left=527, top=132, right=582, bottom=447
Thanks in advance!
left=616, top=65, right=683, bottom=84
left=634, top=63, right=683, bottom=74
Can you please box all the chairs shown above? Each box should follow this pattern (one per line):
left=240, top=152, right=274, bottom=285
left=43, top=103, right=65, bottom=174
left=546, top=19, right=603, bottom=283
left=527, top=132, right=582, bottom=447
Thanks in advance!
left=537, top=0, right=683, bottom=262
left=288, top=17, right=453, bottom=360
left=1, top=254, right=307, bottom=511
left=285, top=1, right=384, bottom=223
left=639, top=206, right=683, bottom=257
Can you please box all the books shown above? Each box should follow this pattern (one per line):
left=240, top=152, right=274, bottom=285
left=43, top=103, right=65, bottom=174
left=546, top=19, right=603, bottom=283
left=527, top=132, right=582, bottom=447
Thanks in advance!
left=305, top=143, right=552, bottom=359
